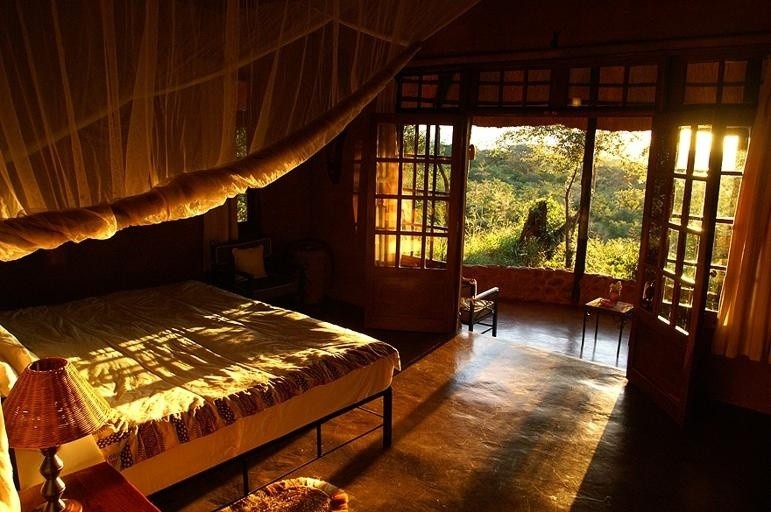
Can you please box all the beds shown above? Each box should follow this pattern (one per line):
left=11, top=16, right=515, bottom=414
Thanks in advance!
left=0, top=278, right=403, bottom=497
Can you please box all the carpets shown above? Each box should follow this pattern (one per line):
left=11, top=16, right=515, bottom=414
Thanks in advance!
left=220, top=476, right=349, bottom=512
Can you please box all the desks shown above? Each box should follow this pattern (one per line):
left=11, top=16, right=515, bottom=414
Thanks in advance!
left=292, top=237, right=334, bottom=306
left=582, top=298, right=636, bottom=358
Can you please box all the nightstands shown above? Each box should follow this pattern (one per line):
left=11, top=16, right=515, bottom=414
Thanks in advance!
left=19, top=461, right=162, bottom=512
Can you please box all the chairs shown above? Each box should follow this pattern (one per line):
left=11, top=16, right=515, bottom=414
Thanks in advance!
left=208, top=234, right=305, bottom=310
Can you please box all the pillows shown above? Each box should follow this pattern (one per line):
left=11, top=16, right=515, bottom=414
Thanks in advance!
left=1, top=324, right=41, bottom=376
left=232, top=245, right=269, bottom=282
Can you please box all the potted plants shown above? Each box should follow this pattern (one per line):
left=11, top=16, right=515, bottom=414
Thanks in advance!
left=609, top=281, right=624, bottom=304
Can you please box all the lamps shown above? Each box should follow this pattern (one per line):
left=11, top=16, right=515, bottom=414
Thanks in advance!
left=3, top=358, right=114, bottom=512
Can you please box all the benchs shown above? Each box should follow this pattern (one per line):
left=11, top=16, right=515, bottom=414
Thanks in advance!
left=392, top=251, right=500, bottom=337
